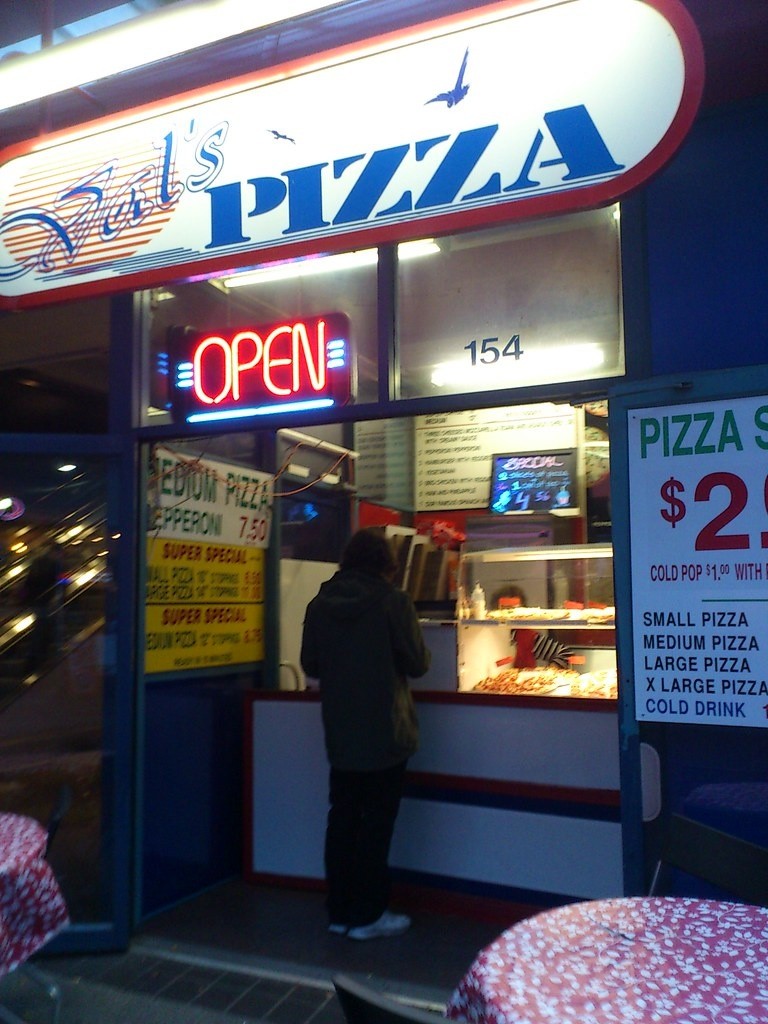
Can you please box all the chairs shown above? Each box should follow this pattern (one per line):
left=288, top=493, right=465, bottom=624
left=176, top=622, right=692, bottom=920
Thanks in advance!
left=330, top=974, right=460, bottom=1024
left=644, top=814, right=768, bottom=907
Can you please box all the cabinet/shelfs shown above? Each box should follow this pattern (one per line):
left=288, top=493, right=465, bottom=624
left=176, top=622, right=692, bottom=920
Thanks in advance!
left=456, top=534, right=623, bottom=710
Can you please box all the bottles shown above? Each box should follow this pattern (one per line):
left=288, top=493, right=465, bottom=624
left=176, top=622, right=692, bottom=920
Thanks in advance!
left=470, top=583, right=485, bottom=620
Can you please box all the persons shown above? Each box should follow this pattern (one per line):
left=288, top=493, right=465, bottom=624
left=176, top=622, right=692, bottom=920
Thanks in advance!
left=299, top=533, right=430, bottom=937
left=511, top=629, right=574, bottom=670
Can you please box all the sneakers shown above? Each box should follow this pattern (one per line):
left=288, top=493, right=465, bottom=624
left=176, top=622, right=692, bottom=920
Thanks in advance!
left=327, top=921, right=348, bottom=934
left=348, top=909, right=411, bottom=941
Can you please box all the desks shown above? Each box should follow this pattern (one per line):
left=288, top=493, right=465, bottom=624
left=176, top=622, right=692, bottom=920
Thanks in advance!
left=446, top=894, right=768, bottom=1024
left=0, top=812, right=72, bottom=1024
